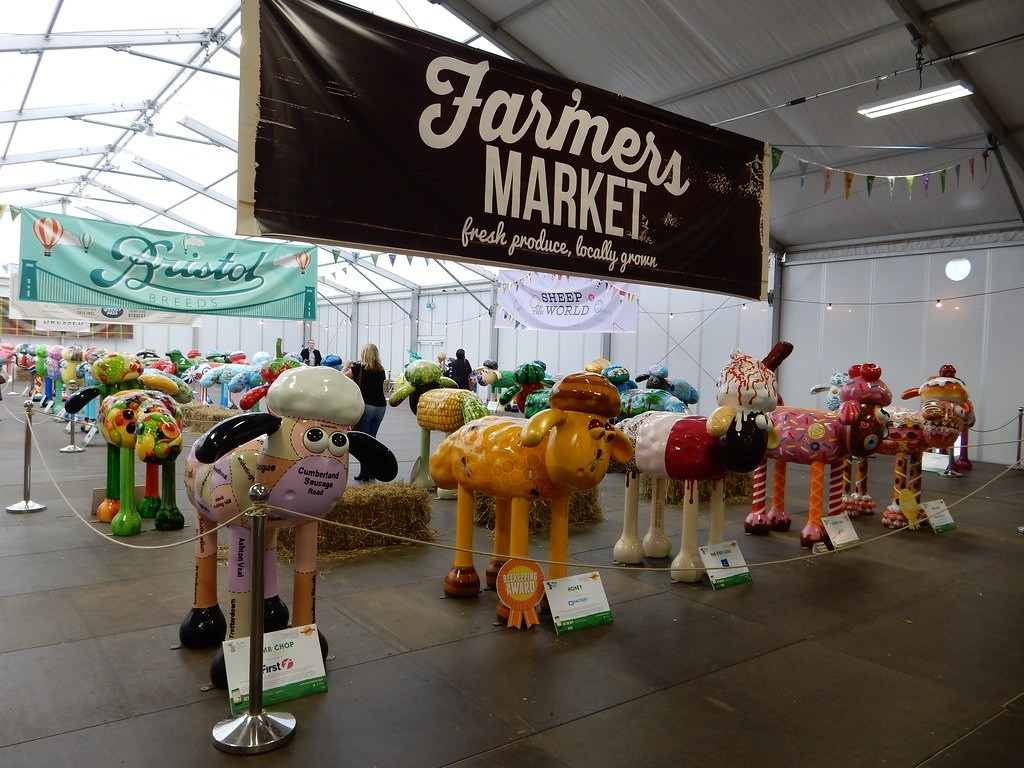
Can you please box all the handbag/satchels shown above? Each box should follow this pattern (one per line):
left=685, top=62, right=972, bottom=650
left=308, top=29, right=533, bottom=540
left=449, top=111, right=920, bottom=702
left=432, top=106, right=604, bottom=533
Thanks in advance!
left=351, top=422, right=359, bottom=431
left=468, top=378, right=473, bottom=391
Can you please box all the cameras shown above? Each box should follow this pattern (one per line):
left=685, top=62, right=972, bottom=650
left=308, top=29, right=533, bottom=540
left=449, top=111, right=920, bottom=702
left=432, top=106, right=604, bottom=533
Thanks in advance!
left=352, top=361, right=358, bottom=366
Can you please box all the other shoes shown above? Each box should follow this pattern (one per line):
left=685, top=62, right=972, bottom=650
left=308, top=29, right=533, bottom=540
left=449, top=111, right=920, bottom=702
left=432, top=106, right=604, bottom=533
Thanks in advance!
left=354, top=475, right=368, bottom=482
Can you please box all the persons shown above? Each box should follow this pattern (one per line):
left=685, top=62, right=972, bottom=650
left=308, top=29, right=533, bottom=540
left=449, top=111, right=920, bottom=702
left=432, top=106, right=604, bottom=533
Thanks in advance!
left=0, top=354, right=13, bottom=421
left=300, top=340, right=322, bottom=366
left=341, top=344, right=387, bottom=481
left=452, top=349, right=472, bottom=390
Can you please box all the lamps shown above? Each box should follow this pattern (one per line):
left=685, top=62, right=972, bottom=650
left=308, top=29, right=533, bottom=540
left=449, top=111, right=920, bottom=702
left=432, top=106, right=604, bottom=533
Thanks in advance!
left=141, top=122, right=156, bottom=137
left=857, top=44, right=974, bottom=119
